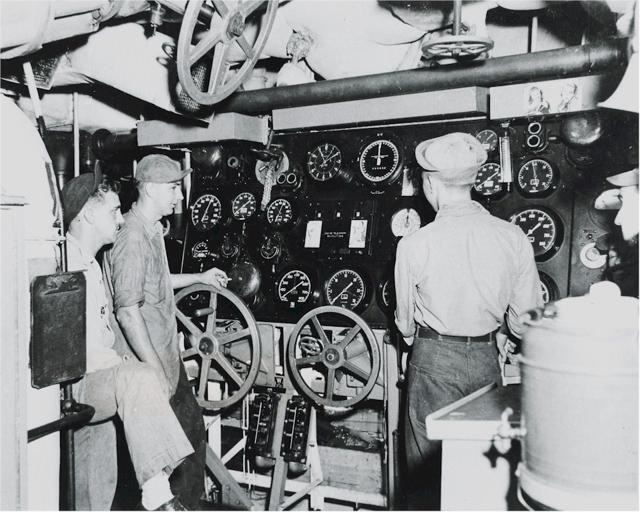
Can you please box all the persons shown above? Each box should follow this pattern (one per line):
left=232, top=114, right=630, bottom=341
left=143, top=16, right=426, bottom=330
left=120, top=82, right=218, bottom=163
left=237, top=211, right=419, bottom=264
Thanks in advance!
left=395, top=130, right=543, bottom=511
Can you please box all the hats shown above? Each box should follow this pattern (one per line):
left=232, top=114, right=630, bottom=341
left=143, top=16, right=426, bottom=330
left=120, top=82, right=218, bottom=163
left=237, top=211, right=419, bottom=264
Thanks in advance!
left=60, top=159, right=103, bottom=233
left=415, top=131, right=488, bottom=172
left=135, top=153, right=194, bottom=183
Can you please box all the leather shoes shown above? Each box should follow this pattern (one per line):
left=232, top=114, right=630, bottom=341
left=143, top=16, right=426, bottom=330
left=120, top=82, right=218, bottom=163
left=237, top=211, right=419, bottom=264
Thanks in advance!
left=135, top=496, right=190, bottom=511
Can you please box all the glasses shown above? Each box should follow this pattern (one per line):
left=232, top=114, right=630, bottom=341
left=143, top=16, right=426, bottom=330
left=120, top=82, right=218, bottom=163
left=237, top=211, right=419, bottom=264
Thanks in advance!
left=98, top=180, right=122, bottom=193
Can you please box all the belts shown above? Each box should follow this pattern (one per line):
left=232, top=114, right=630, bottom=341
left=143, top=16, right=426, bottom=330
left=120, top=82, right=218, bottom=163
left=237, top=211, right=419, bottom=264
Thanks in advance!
left=418, top=328, right=496, bottom=342
